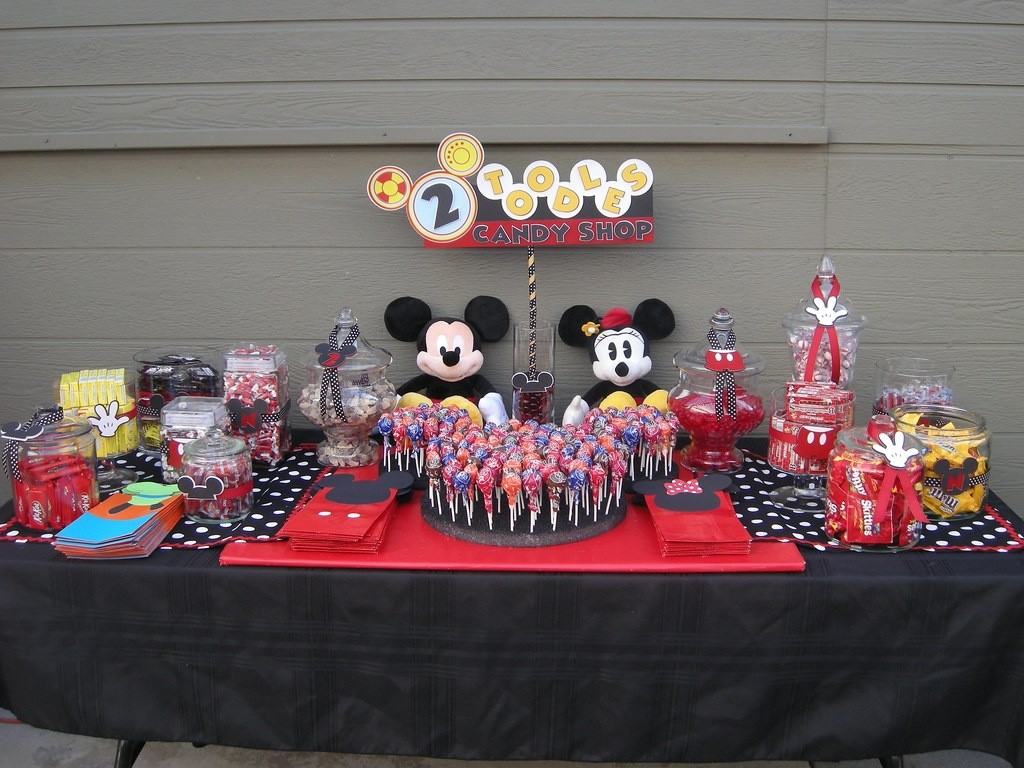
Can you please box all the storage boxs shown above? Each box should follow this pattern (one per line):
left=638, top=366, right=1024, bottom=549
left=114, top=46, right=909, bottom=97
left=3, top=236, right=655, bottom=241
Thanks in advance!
left=59, top=368, right=139, bottom=458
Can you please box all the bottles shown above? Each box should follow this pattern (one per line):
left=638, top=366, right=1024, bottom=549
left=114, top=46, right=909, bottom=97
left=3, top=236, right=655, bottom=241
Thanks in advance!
left=296, top=306, right=397, bottom=469
left=823, top=424, right=926, bottom=553
left=181, top=428, right=255, bottom=524
left=888, top=403, right=993, bottom=523
left=131, top=345, right=222, bottom=459
left=223, top=340, right=295, bottom=468
left=9, top=402, right=99, bottom=534
left=160, top=395, right=232, bottom=486
left=666, top=307, right=767, bottom=476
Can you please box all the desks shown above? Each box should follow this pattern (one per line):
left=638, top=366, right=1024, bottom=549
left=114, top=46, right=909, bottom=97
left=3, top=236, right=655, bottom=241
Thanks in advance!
left=0, top=428, right=1024, bottom=768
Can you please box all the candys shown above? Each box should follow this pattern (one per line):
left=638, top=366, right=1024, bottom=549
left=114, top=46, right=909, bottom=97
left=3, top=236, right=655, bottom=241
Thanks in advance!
left=378, top=402, right=678, bottom=536
left=296, top=376, right=395, bottom=467
left=768, top=334, right=988, bottom=547
left=10, top=451, right=98, bottom=532
left=135, top=343, right=289, bottom=522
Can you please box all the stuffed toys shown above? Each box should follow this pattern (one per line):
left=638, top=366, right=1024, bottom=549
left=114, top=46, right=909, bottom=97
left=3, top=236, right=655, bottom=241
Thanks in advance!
left=384, top=296, right=510, bottom=427
left=559, top=298, right=675, bottom=429
